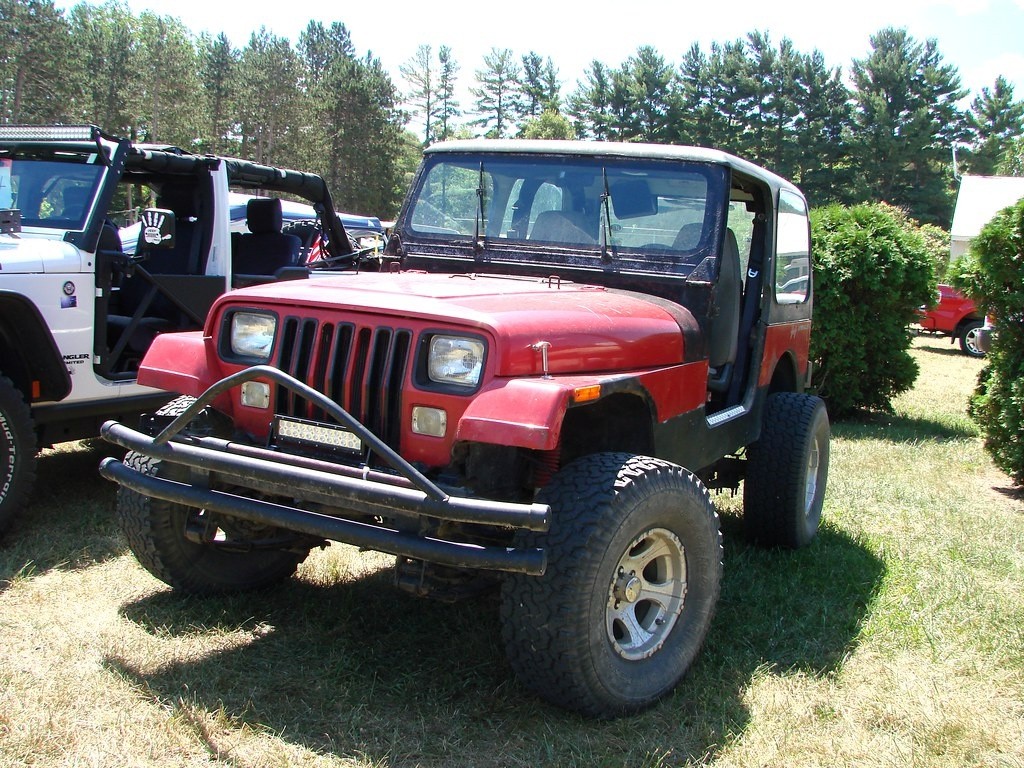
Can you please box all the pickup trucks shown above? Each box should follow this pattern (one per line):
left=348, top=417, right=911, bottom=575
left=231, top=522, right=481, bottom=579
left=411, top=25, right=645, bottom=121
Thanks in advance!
left=916, top=284, right=989, bottom=359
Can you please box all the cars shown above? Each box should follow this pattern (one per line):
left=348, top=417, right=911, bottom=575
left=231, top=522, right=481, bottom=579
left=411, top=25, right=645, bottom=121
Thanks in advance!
left=117, top=192, right=382, bottom=256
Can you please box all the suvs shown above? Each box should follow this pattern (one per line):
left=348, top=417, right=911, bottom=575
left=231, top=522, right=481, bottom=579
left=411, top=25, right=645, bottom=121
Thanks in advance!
left=98, top=139, right=831, bottom=720
left=0, top=125, right=381, bottom=537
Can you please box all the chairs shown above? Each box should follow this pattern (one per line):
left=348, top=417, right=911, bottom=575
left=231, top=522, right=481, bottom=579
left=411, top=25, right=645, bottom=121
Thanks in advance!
left=528, top=211, right=593, bottom=245
left=103, top=194, right=301, bottom=367
left=671, top=223, right=742, bottom=392
left=62, top=186, right=121, bottom=290
